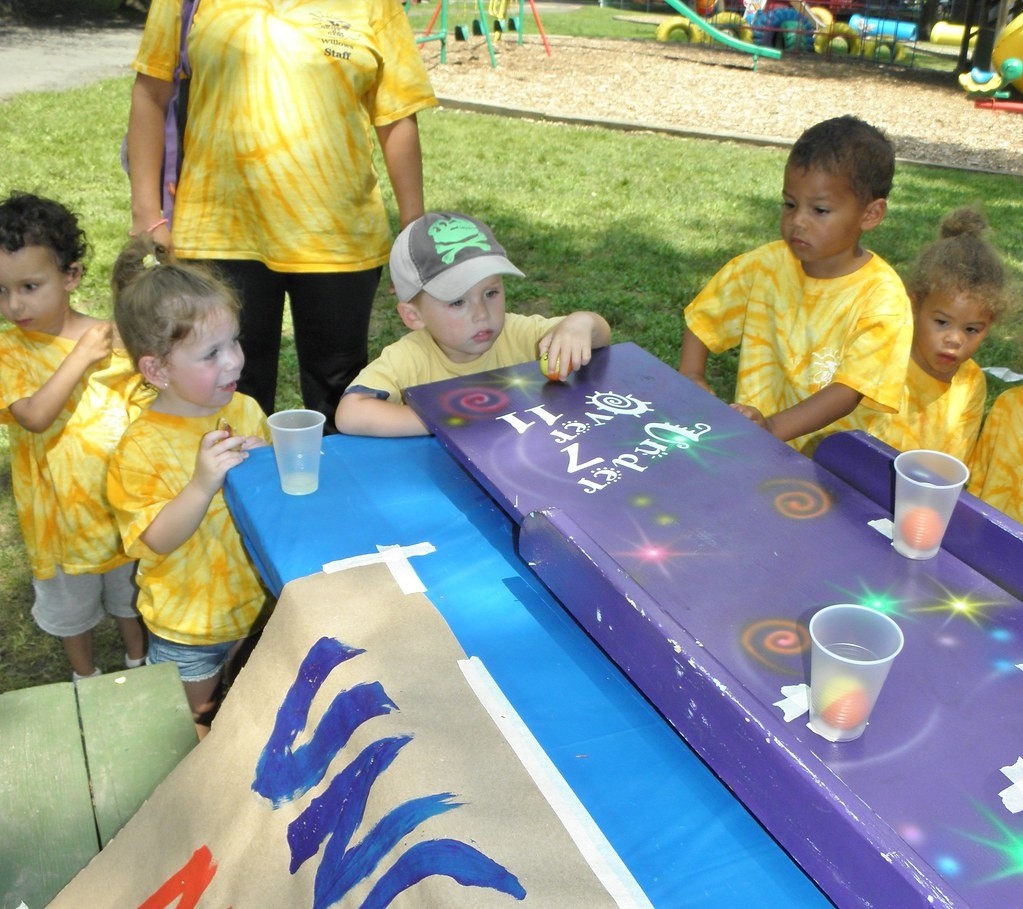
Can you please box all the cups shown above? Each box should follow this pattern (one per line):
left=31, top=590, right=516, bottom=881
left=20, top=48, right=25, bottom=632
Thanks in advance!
left=267, top=408, right=326, bottom=496
left=893, top=449, right=971, bottom=560
left=809, top=605, right=905, bottom=741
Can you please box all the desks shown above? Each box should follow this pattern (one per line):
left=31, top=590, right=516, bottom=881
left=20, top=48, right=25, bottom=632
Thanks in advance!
left=223, top=431, right=1022, bottom=909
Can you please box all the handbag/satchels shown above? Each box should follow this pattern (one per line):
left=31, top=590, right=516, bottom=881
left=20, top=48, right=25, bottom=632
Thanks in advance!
left=120, top=0, right=201, bottom=230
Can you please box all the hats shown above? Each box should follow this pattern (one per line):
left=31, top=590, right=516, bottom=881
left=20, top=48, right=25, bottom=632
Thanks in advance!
left=389, top=210, right=526, bottom=303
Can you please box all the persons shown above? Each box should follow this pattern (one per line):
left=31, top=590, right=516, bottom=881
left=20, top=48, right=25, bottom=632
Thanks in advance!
left=0, top=193, right=157, bottom=680
left=335, top=210, right=613, bottom=436
left=869, top=210, right=1003, bottom=481
left=678, top=114, right=915, bottom=456
left=965, top=385, right=1023, bottom=527
left=108, top=236, right=280, bottom=743
left=127, top=0, right=437, bottom=433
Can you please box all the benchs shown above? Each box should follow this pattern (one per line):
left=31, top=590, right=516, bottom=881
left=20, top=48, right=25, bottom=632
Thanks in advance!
left=0, top=662, right=201, bottom=909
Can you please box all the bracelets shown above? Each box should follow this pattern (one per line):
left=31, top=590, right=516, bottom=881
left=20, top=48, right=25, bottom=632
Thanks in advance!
left=128, top=218, right=168, bottom=237
